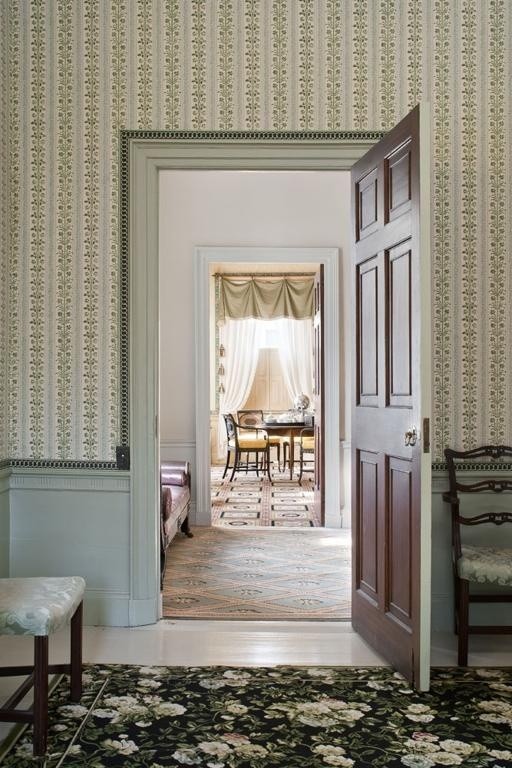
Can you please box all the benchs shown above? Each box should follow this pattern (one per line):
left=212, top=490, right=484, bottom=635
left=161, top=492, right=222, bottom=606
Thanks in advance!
left=160, top=461, right=193, bottom=586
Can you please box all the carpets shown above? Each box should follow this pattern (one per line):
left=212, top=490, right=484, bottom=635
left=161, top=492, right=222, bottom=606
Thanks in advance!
left=0, top=664, right=512, bottom=768
left=159, top=528, right=353, bottom=621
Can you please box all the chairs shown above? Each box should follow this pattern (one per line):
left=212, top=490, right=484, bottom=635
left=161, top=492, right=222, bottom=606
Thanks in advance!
left=443, top=445, right=512, bottom=669
left=0, top=578, right=84, bottom=754
left=221, top=410, right=315, bottom=486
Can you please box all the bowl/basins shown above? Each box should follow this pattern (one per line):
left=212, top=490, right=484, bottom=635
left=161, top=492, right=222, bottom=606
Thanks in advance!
left=264, top=412, right=298, bottom=424
